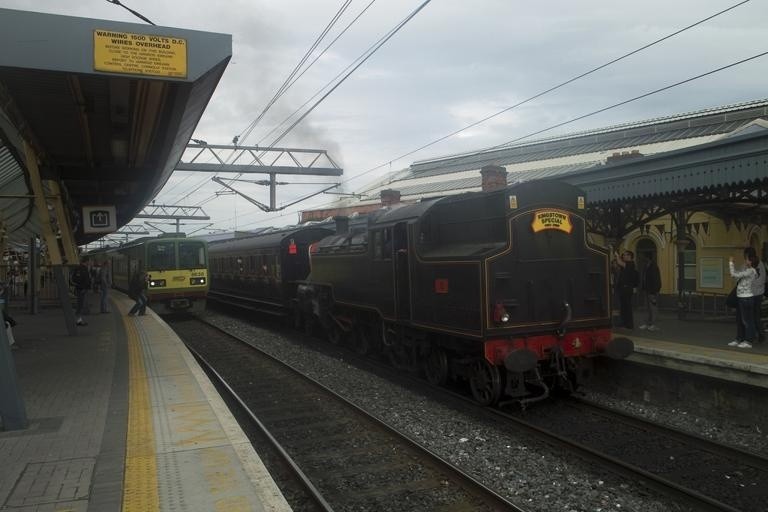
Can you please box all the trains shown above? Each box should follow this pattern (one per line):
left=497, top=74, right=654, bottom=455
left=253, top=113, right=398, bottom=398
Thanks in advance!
left=192, top=163, right=637, bottom=412
left=78, top=232, right=212, bottom=319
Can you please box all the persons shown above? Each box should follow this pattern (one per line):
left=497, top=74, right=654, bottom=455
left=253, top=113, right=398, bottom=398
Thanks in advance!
left=71, top=255, right=112, bottom=326
left=740, top=247, right=766, bottom=344
left=727, top=255, right=760, bottom=349
left=610, top=250, right=662, bottom=332
left=127, top=271, right=149, bottom=317
left=4, top=311, right=20, bottom=351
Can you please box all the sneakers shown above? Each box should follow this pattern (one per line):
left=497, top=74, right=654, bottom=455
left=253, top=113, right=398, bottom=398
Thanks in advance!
left=728, top=341, right=752, bottom=349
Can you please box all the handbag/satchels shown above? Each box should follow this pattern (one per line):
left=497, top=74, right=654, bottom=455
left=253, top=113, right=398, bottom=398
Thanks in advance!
left=725, top=288, right=739, bottom=309
